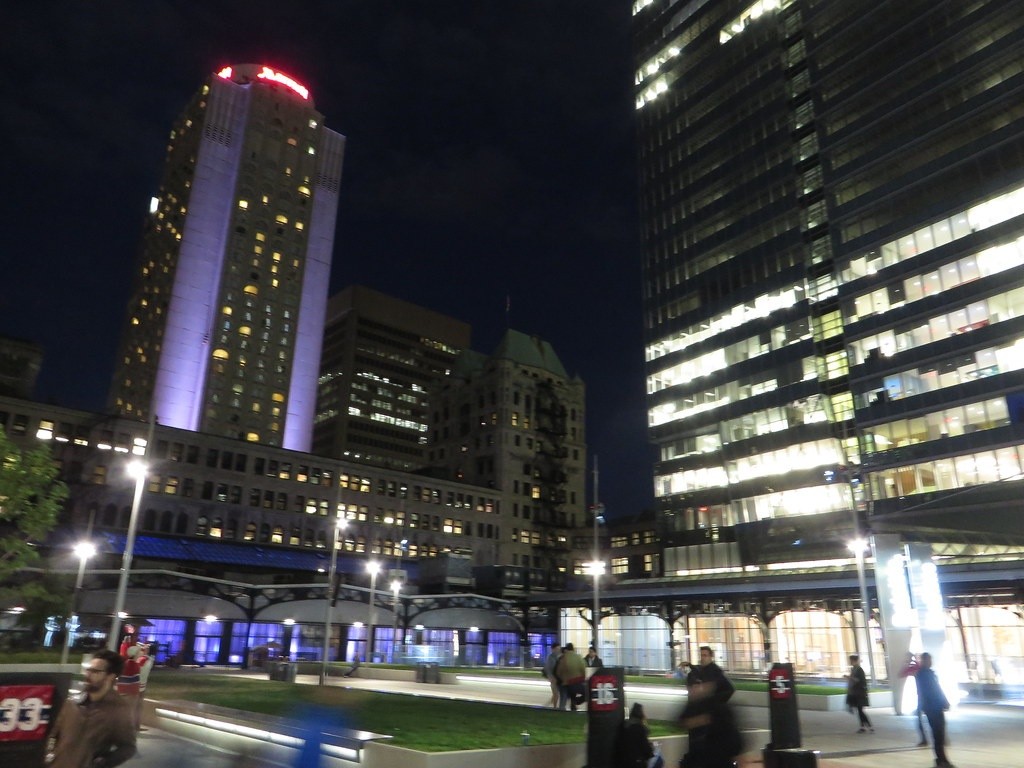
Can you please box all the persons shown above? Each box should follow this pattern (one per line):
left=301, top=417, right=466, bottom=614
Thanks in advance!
left=544, top=642, right=605, bottom=712
left=842, top=656, right=875, bottom=735
left=344, top=651, right=361, bottom=676
left=897, top=651, right=954, bottom=768
left=41, top=648, right=136, bottom=768
left=505, top=649, right=512, bottom=666
left=613, top=703, right=664, bottom=768
left=675, top=646, right=747, bottom=768
left=116, top=634, right=157, bottom=743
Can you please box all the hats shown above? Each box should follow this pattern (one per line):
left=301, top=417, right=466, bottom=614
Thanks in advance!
left=127, top=646, right=144, bottom=656
left=629, top=702, right=646, bottom=720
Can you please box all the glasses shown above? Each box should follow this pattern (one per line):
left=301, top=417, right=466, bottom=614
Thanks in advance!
left=86, top=668, right=106, bottom=674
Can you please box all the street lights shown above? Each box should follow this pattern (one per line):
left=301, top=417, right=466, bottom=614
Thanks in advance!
left=102, top=457, right=149, bottom=651
left=59, top=540, right=97, bottom=664
left=390, top=579, right=402, bottom=663
left=365, top=559, right=382, bottom=662
left=588, top=560, right=605, bottom=659
left=317, top=517, right=351, bottom=686
left=848, top=538, right=878, bottom=688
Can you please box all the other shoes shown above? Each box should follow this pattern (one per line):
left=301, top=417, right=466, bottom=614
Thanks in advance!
left=936, top=759, right=955, bottom=768
left=857, top=728, right=874, bottom=734
left=918, top=742, right=927, bottom=747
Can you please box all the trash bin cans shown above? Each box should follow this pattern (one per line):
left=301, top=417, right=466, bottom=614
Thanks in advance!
left=415, top=660, right=440, bottom=684
left=270, top=662, right=296, bottom=683
